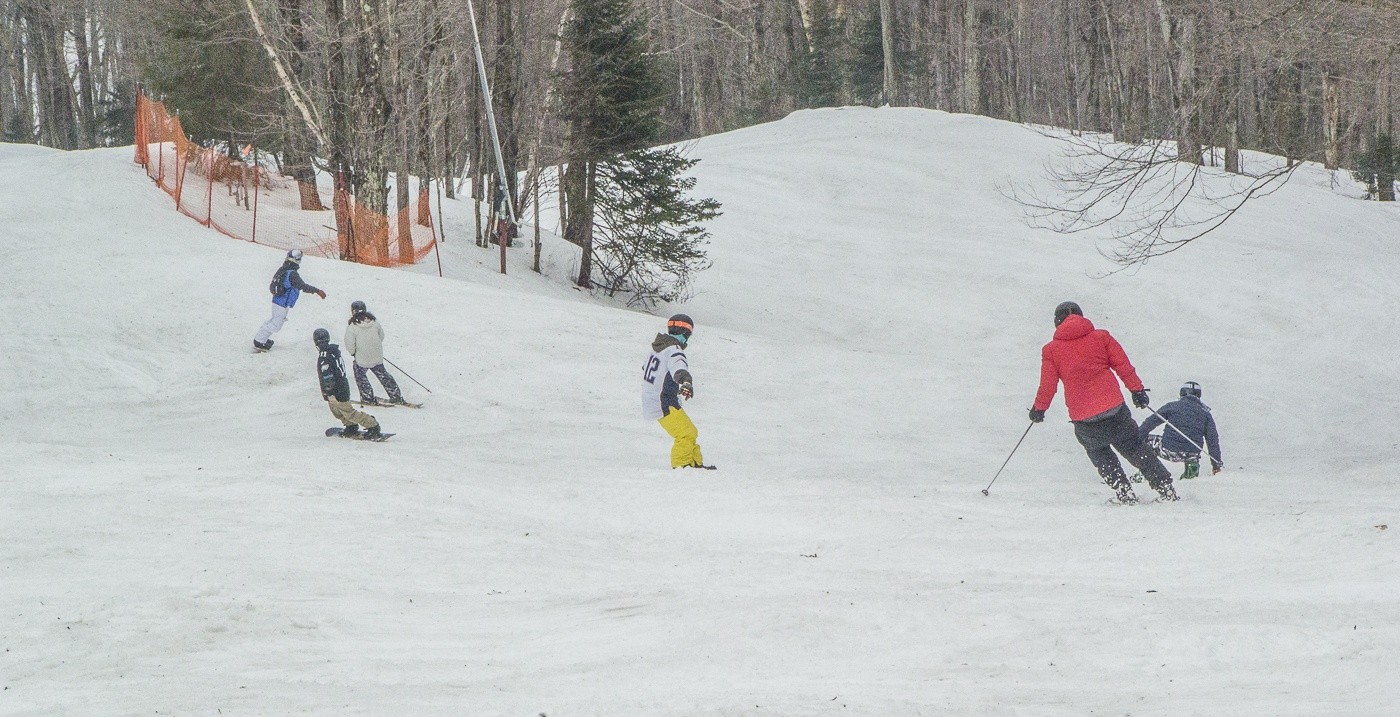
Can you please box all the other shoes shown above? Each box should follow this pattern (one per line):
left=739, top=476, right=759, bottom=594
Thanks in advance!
left=253, top=340, right=265, bottom=349
left=264, top=339, right=274, bottom=350
left=361, top=416, right=380, bottom=439
left=361, top=398, right=378, bottom=405
left=340, top=423, right=359, bottom=438
left=390, top=397, right=406, bottom=405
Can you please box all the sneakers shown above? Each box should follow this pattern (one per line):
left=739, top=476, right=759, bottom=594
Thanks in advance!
left=1178, top=461, right=1200, bottom=480
left=1117, top=483, right=1140, bottom=506
left=1130, top=472, right=1146, bottom=483
left=1155, top=478, right=1179, bottom=504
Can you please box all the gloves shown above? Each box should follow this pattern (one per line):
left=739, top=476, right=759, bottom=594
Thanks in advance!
left=1212, top=465, right=1221, bottom=474
left=327, top=395, right=339, bottom=406
left=1132, top=385, right=1150, bottom=409
left=678, top=376, right=694, bottom=401
left=315, top=288, right=326, bottom=299
left=1028, top=405, right=1046, bottom=423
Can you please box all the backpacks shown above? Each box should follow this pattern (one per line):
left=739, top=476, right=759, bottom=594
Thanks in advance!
left=270, top=267, right=293, bottom=296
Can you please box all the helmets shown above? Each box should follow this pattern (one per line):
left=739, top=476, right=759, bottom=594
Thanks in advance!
left=1054, top=302, right=1083, bottom=329
left=667, top=314, right=694, bottom=342
left=350, top=301, right=366, bottom=316
left=1180, top=381, right=1201, bottom=398
left=312, top=328, right=331, bottom=346
left=286, top=249, right=302, bottom=264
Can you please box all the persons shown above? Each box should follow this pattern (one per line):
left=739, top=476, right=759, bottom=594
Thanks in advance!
left=1029, top=301, right=1179, bottom=504
left=642, top=313, right=717, bottom=471
left=254, top=250, right=326, bottom=349
left=313, top=328, right=380, bottom=440
left=1129, top=381, right=1221, bottom=482
left=345, top=301, right=407, bottom=406
